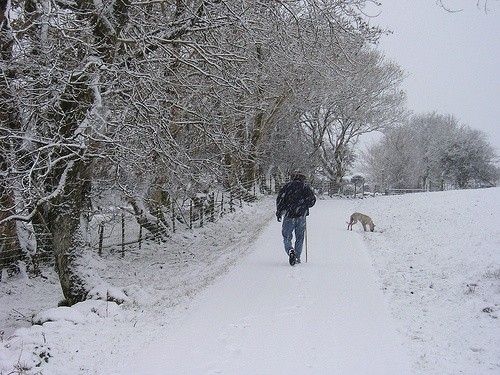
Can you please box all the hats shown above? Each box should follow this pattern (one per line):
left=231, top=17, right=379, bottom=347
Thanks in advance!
left=291, top=170, right=306, bottom=181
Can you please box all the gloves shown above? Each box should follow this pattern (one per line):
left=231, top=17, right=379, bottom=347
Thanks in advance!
left=277, top=216, right=281, bottom=222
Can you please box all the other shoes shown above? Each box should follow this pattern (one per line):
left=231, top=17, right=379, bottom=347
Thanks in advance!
left=289, top=250, right=296, bottom=265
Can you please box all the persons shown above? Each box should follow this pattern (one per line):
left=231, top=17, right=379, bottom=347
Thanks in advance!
left=275, top=171, right=316, bottom=266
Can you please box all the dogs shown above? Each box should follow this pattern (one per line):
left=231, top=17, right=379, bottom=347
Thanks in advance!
left=345, top=211, right=376, bottom=232
